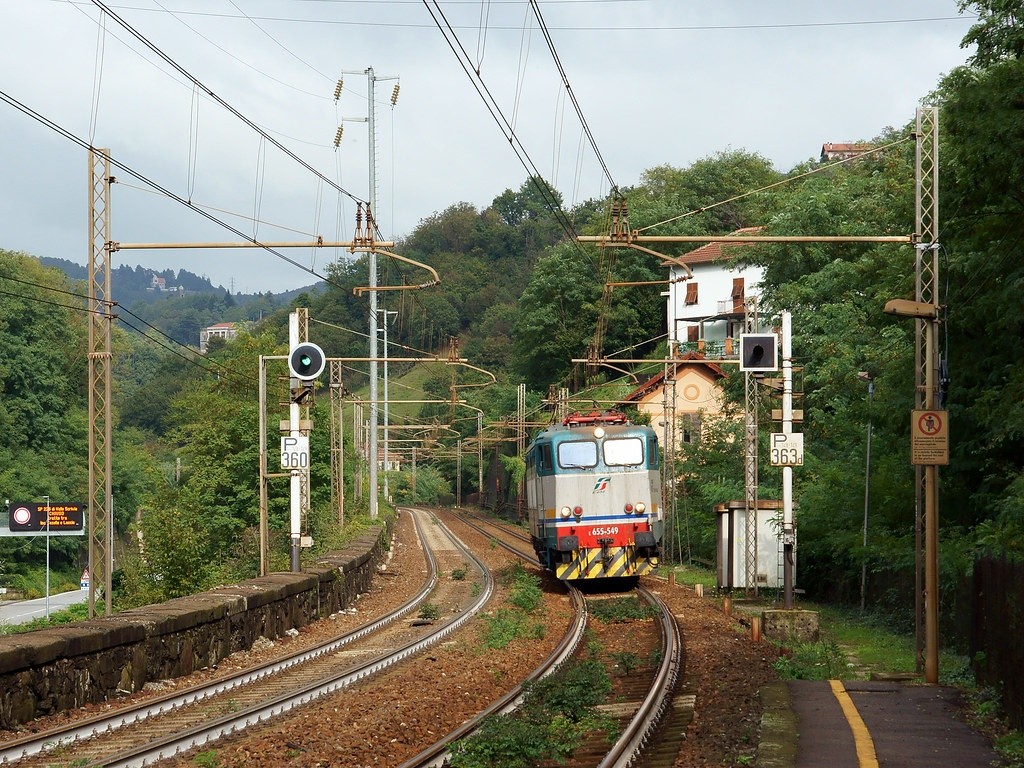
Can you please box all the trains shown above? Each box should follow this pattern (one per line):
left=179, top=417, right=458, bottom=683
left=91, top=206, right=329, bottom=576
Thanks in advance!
left=524, top=405, right=666, bottom=581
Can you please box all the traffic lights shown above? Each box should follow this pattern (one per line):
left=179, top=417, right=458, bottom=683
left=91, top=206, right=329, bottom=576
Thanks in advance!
left=743, top=336, right=774, bottom=368
left=292, top=346, right=322, bottom=376
